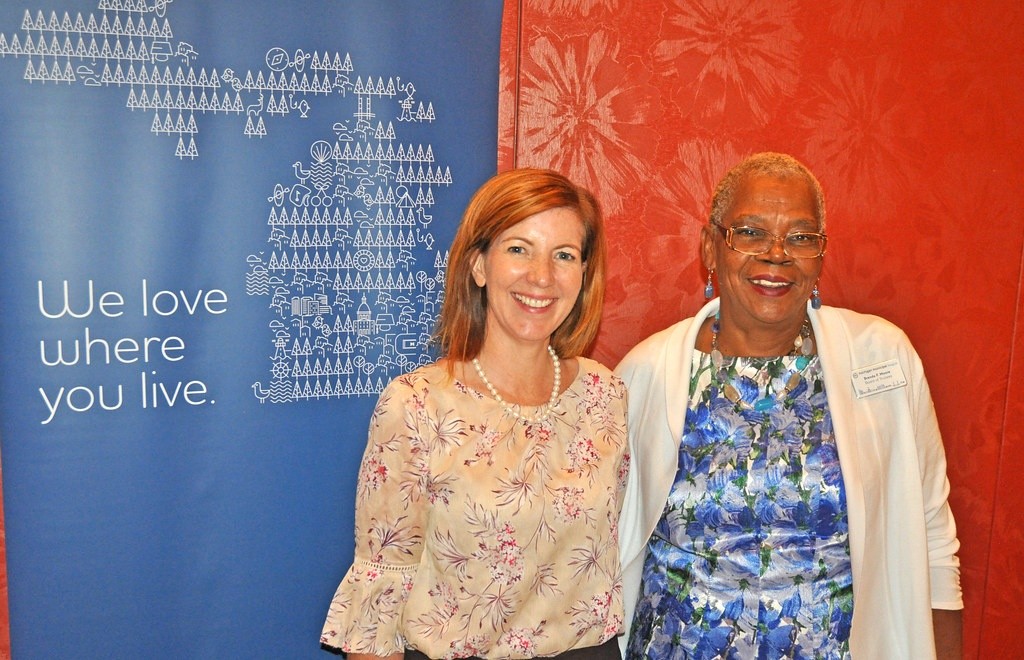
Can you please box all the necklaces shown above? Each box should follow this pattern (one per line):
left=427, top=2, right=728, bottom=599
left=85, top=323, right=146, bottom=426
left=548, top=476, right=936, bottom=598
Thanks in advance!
left=472, top=346, right=561, bottom=423
left=706, top=315, right=812, bottom=411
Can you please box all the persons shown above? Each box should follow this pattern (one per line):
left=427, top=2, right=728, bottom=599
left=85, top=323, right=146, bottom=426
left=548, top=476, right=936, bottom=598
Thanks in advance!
left=317, top=167, right=630, bottom=660
left=612, top=151, right=966, bottom=660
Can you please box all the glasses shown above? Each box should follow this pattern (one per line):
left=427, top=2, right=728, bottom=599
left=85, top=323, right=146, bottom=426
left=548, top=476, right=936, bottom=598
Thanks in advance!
left=711, top=219, right=828, bottom=260
